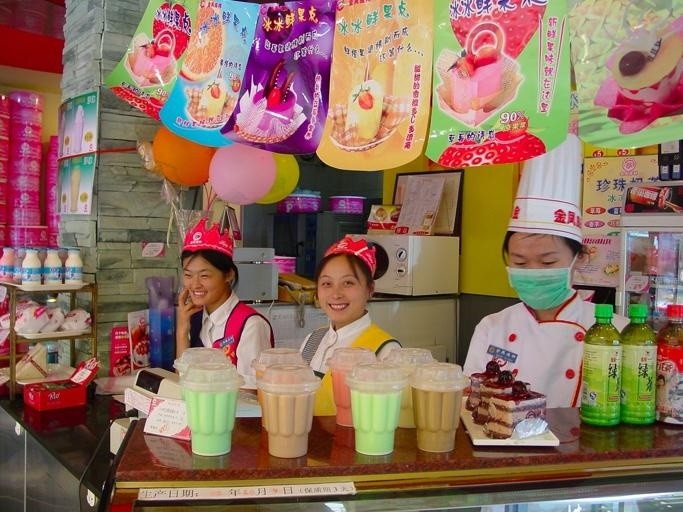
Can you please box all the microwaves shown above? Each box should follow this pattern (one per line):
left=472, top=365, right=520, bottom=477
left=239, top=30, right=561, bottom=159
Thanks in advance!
left=343, top=230, right=460, bottom=297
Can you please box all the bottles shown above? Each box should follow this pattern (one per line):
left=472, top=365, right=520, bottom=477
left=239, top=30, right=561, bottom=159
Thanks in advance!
left=43, top=343, right=58, bottom=372
left=657, top=153, right=681, bottom=181
left=619, top=305, right=656, bottom=425
left=657, top=303, right=682, bottom=425
left=0, top=245, right=83, bottom=287
left=580, top=302, right=620, bottom=429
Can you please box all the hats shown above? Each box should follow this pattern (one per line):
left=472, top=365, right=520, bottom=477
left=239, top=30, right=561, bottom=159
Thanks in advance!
left=506, top=133, right=583, bottom=245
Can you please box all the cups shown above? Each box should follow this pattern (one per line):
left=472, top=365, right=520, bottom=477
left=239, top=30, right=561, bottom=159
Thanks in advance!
left=67, top=166, right=81, bottom=212
left=173, top=344, right=468, bottom=457
left=625, top=186, right=673, bottom=209
left=71, top=117, right=84, bottom=153
left=191, top=421, right=458, bottom=465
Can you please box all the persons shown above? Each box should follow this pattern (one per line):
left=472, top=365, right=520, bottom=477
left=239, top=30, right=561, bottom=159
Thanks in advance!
left=175, top=216, right=276, bottom=394
left=460, top=226, right=631, bottom=413
left=295, top=233, right=405, bottom=417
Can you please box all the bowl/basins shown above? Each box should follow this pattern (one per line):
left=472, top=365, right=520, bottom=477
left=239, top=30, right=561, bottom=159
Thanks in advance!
left=185, top=109, right=226, bottom=129
left=234, top=128, right=296, bottom=144
left=326, top=125, right=399, bottom=153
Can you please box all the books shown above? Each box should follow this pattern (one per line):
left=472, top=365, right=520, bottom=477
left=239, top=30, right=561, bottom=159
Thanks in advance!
left=460, top=413, right=560, bottom=446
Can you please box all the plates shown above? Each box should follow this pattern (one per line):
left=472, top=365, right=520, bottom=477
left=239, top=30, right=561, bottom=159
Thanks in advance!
left=459, top=394, right=561, bottom=447
left=113, top=367, right=130, bottom=376
left=132, top=359, right=151, bottom=367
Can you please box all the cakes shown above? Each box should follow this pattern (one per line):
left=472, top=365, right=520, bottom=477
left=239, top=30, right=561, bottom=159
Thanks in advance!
left=432, top=23, right=523, bottom=127
left=465, top=360, right=547, bottom=441
left=133, top=340, right=151, bottom=367
left=185, top=81, right=237, bottom=129
left=122, top=29, right=178, bottom=88
left=14, top=341, right=47, bottom=379
left=326, top=61, right=404, bottom=153
left=230, top=60, right=306, bottom=144
left=594, top=16, right=682, bottom=134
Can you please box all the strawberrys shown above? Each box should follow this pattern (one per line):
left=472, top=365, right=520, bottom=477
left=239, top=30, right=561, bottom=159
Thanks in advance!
left=438, top=130, right=545, bottom=166
left=450, top=0, right=545, bottom=62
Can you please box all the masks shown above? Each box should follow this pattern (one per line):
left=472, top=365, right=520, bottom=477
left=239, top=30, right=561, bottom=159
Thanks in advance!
left=505, top=253, right=579, bottom=311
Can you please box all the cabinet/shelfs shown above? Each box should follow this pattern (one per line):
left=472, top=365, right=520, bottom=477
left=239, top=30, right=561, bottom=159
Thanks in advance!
left=105, top=402, right=683, bottom=511
left=0, top=394, right=120, bottom=512
left=251, top=296, right=461, bottom=364
left=619, top=211, right=683, bottom=321
left=0, top=247, right=100, bottom=405
left=269, top=210, right=371, bottom=278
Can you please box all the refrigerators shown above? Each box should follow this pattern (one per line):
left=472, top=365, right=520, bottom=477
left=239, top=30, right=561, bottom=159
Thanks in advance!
left=617, top=181, right=682, bottom=330
